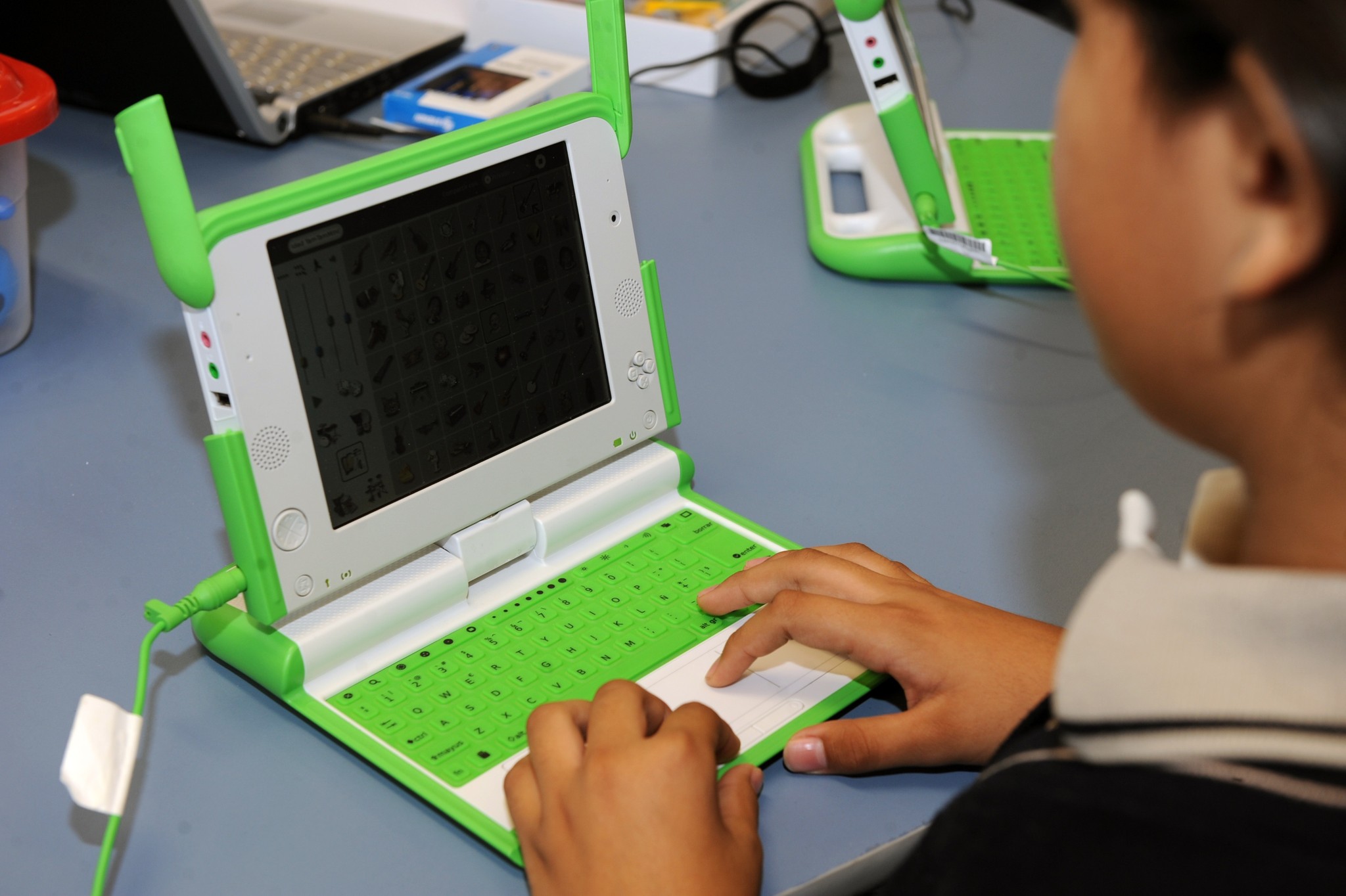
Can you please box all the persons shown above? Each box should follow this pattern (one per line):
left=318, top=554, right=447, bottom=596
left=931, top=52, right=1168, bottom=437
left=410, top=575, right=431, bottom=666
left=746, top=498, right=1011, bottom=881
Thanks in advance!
left=504, top=0, right=1346, bottom=896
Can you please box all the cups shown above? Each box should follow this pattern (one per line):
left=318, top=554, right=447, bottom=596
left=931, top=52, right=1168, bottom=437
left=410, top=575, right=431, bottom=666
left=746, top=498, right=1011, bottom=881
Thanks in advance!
left=0, top=53, right=59, bottom=357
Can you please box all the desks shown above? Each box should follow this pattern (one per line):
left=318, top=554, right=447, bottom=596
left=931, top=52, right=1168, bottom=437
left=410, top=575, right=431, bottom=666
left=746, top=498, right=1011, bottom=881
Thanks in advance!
left=0, top=0, right=1229, bottom=896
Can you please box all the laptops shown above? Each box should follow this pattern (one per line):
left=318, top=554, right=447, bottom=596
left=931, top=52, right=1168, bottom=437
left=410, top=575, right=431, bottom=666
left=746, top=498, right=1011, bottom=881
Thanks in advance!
left=111, top=1, right=926, bottom=876
left=0, top=0, right=472, bottom=154
left=797, top=0, right=1076, bottom=291
